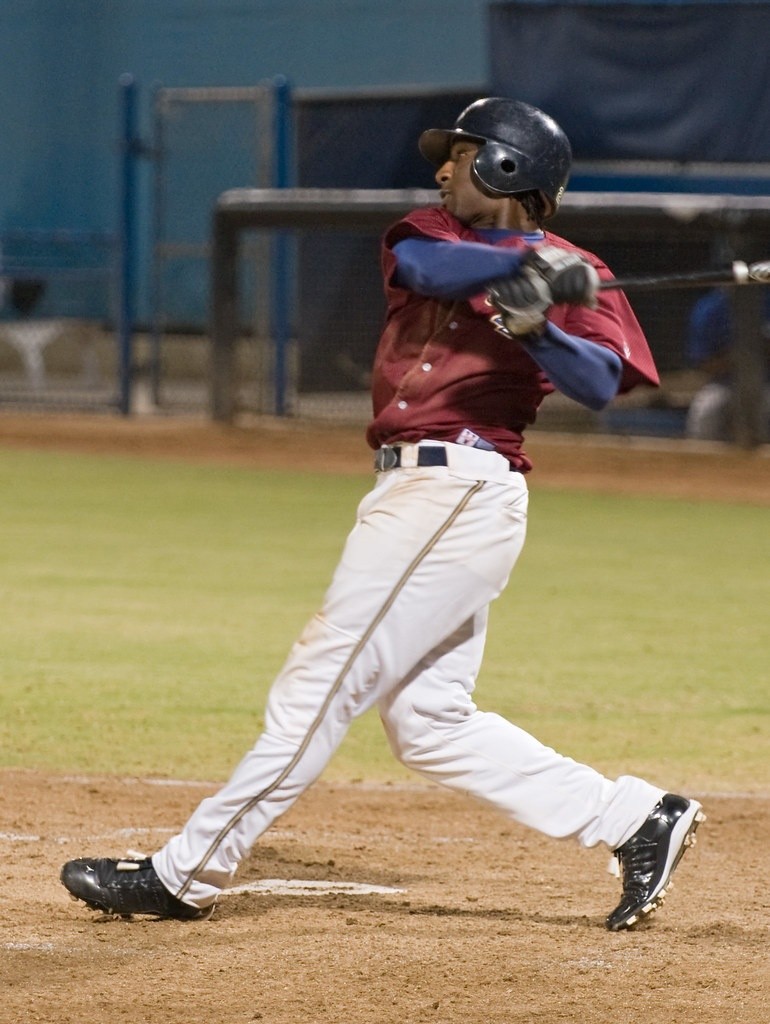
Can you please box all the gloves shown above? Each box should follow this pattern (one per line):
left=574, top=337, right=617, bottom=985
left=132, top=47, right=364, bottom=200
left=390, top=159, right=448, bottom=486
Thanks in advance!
left=522, top=244, right=600, bottom=312
left=485, top=264, right=554, bottom=340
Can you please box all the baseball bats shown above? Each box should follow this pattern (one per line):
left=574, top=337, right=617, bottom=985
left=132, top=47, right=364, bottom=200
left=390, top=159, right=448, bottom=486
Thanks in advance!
left=491, top=259, right=770, bottom=306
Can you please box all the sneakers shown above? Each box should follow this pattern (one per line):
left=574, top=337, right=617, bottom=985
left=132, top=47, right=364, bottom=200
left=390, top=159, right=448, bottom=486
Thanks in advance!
left=60, top=852, right=218, bottom=924
left=604, top=792, right=707, bottom=930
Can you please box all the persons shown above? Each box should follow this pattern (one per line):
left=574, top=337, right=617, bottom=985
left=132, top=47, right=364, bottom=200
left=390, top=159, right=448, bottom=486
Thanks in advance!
left=61, top=98, right=707, bottom=935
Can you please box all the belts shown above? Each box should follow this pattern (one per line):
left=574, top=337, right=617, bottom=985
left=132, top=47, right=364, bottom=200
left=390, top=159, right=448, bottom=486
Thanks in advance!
left=373, top=445, right=519, bottom=472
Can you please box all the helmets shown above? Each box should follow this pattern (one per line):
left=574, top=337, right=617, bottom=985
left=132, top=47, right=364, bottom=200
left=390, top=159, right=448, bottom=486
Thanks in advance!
left=419, top=96, right=574, bottom=219
